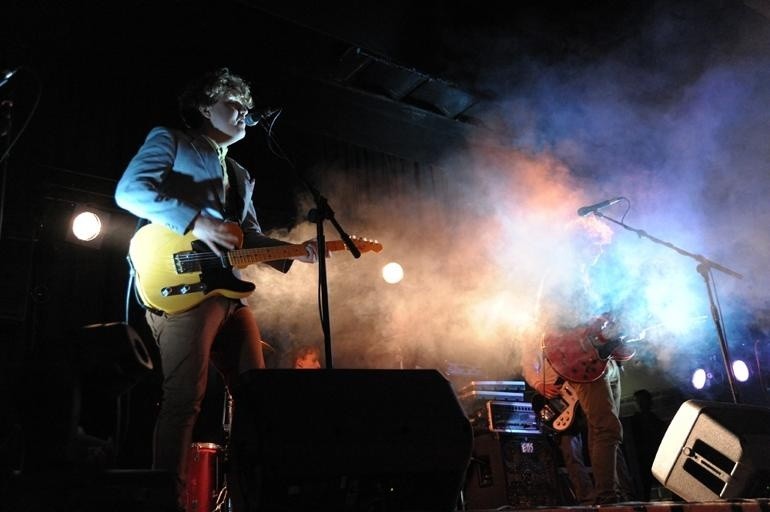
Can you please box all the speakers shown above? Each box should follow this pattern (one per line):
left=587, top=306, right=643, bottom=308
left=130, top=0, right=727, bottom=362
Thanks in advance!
left=651, top=398, right=770, bottom=505
left=226, top=369, right=474, bottom=512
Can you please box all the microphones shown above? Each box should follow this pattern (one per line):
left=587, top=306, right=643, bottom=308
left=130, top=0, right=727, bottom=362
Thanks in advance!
left=577, top=196, right=624, bottom=217
left=245, top=103, right=283, bottom=127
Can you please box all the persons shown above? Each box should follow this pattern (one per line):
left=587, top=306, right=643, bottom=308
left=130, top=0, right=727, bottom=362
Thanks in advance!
left=541, top=219, right=629, bottom=506
left=111, top=64, right=334, bottom=512
left=276, top=335, right=323, bottom=368
left=623, top=388, right=676, bottom=499
left=509, top=300, right=596, bottom=507
left=361, top=334, right=406, bottom=368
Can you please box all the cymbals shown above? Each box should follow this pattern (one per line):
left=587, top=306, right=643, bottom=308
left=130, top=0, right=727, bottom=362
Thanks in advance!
left=259, top=339, right=275, bottom=354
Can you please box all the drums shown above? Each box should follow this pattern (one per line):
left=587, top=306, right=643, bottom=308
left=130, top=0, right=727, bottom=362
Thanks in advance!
left=183, top=442, right=224, bottom=510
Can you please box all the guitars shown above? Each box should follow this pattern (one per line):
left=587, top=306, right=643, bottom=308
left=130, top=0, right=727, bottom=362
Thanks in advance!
left=540, top=306, right=652, bottom=383
left=129, top=223, right=383, bottom=315
